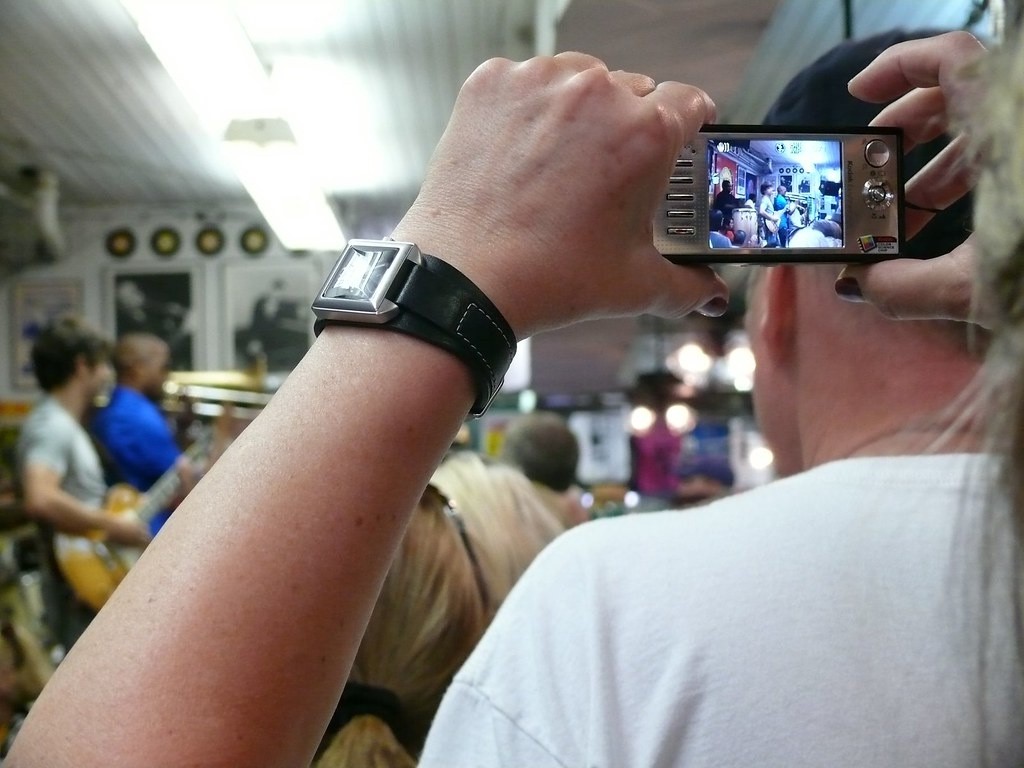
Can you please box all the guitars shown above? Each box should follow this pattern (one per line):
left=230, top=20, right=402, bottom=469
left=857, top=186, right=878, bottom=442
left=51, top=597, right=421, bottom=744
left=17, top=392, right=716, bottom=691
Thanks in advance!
left=766, top=202, right=790, bottom=232
left=52, top=404, right=236, bottom=613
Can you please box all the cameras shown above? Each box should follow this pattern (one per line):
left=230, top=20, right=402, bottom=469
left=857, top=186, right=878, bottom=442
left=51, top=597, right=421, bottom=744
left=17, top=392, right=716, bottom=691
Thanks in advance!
left=651, top=124, right=906, bottom=266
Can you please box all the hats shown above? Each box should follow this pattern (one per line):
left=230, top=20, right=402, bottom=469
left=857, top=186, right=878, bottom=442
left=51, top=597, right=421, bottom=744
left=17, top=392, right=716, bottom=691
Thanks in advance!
left=763, top=26, right=972, bottom=259
left=761, top=180, right=772, bottom=186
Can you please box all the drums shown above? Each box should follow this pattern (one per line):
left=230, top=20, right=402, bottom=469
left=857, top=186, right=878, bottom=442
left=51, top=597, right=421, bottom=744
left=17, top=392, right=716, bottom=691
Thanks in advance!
left=732, top=207, right=758, bottom=243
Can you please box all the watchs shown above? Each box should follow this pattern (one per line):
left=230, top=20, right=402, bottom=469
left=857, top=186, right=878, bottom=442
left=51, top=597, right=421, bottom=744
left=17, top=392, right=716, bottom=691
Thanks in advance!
left=312, top=236, right=517, bottom=422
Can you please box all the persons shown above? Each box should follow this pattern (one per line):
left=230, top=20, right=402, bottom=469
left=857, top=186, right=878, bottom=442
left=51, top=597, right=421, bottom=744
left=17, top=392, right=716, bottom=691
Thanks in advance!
left=413, top=28, right=1024, bottom=768
left=0, top=326, right=735, bottom=768
left=709, top=180, right=843, bottom=247
left=0, top=0, right=1024, bottom=768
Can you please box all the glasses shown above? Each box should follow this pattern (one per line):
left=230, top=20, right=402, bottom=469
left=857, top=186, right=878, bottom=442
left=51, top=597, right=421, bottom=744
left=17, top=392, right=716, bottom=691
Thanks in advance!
left=426, top=483, right=486, bottom=613
